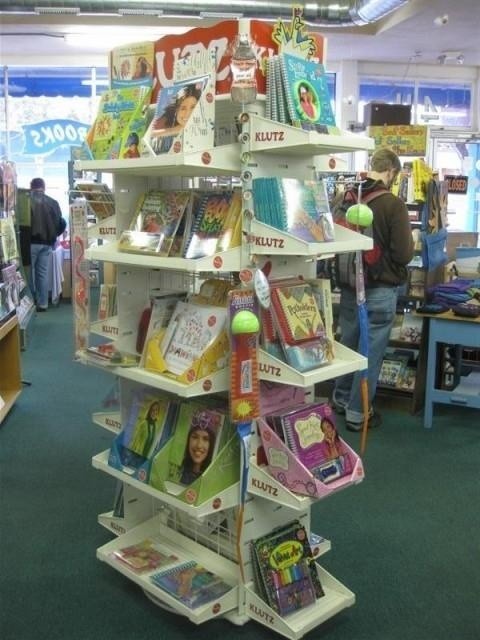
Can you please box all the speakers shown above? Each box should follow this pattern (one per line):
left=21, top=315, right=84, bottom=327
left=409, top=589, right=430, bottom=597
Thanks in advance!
left=387, top=104, right=411, bottom=125
left=363, top=103, right=388, bottom=127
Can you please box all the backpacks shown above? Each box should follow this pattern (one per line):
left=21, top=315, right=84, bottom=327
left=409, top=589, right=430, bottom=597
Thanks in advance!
left=322, top=181, right=393, bottom=294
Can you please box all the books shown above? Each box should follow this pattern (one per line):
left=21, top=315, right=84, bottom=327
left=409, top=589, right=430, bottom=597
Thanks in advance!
left=77, top=40, right=341, bottom=611
left=376, top=157, right=452, bottom=392
left=0, top=160, right=25, bottom=322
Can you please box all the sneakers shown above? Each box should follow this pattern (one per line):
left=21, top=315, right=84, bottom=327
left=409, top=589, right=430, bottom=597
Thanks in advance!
left=344, top=410, right=384, bottom=434
left=325, top=391, right=349, bottom=415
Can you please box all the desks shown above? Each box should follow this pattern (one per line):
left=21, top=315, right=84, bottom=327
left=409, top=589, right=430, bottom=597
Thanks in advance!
left=415, top=304, right=480, bottom=429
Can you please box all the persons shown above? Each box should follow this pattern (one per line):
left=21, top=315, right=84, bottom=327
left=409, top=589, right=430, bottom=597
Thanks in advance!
left=17, top=176, right=67, bottom=311
left=333, top=148, right=418, bottom=431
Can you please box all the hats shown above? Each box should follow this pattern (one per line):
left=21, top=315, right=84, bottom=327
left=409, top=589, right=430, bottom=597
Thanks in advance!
left=189, top=408, right=218, bottom=436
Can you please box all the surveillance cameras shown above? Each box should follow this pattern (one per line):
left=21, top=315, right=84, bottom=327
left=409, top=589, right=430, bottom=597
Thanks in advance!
left=434, top=15, right=448, bottom=25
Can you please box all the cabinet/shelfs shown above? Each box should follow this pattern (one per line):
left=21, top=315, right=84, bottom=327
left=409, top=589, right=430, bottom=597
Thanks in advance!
left=315, top=170, right=450, bottom=414
